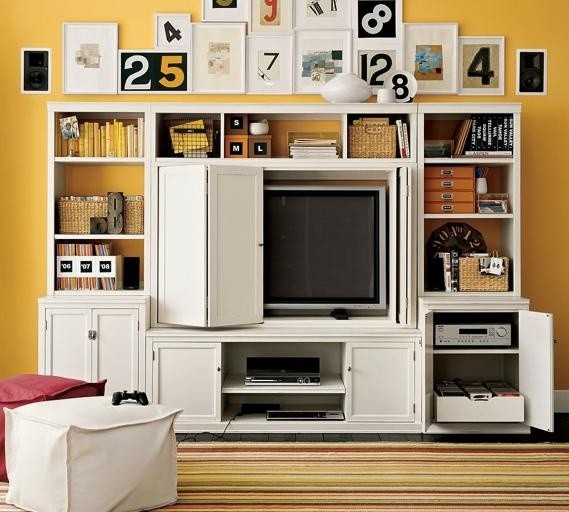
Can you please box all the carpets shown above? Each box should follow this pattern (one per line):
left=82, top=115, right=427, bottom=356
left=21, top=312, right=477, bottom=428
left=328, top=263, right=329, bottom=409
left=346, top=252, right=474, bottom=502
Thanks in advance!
left=1, top=441, right=569, bottom=511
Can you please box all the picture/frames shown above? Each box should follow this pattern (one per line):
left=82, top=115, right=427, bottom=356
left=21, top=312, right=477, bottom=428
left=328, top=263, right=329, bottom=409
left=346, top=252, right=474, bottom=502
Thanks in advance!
left=21, top=48, right=51, bottom=94
left=63, top=22, right=118, bottom=95
left=118, top=49, right=192, bottom=95
left=402, top=23, right=459, bottom=95
left=459, top=36, right=505, bottom=95
left=191, top=0, right=402, bottom=94
left=156, top=13, right=190, bottom=49
left=516, top=49, right=547, bottom=95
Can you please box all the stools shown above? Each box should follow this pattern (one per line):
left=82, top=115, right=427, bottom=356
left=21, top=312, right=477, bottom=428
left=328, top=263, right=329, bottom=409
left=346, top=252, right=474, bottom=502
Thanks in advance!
left=2, top=399, right=183, bottom=512
left=0, top=373, right=107, bottom=483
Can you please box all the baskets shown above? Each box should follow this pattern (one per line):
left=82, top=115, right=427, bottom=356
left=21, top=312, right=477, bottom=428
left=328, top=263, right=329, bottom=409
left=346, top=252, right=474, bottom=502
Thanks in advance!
left=58, top=196, right=144, bottom=235
left=348, top=124, right=397, bottom=159
left=458, top=256, right=509, bottom=292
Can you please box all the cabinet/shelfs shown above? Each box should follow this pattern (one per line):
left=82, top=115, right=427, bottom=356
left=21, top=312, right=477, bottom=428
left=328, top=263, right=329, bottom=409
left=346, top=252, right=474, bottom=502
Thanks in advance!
left=150, top=103, right=419, bottom=433
left=417, top=103, right=553, bottom=434
left=38, top=101, right=150, bottom=396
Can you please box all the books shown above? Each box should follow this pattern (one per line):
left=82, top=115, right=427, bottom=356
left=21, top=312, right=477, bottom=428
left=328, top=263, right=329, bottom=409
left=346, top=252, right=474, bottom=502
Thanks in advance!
left=452, top=114, right=514, bottom=158
left=56, top=242, right=116, bottom=291
left=395, top=118, right=410, bottom=159
left=450, top=250, right=459, bottom=293
left=288, top=137, right=341, bottom=161
left=434, top=252, right=452, bottom=293
left=55, top=116, right=145, bottom=158
left=167, top=117, right=214, bottom=156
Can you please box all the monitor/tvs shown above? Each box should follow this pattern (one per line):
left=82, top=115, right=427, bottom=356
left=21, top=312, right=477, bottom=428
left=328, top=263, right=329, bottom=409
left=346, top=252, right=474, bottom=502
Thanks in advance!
left=263, top=185, right=387, bottom=319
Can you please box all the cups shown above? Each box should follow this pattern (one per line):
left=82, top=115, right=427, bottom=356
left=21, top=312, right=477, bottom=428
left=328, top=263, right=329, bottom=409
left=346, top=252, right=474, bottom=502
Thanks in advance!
left=376, top=88, right=396, bottom=104
left=249, top=123, right=270, bottom=135
left=476, top=177, right=488, bottom=194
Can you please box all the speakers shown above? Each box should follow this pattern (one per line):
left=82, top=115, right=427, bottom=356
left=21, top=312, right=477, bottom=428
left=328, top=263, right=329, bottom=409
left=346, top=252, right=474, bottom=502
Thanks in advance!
left=24, top=51, right=48, bottom=91
left=520, top=52, right=544, bottom=92
